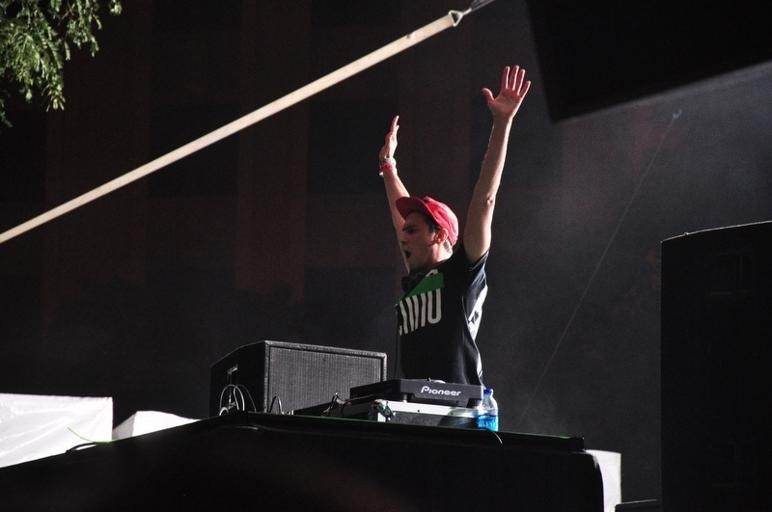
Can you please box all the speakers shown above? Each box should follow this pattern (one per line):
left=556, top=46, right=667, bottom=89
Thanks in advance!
left=209, top=340, right=388, bottom=420
left=659, top=219, right=771, bottom=511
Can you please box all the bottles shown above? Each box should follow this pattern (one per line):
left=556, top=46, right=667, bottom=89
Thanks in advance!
left=478, top=386, right=498, bottom=430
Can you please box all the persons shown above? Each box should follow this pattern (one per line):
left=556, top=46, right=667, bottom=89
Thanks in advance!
left=379, top=65, right=531, bottom=383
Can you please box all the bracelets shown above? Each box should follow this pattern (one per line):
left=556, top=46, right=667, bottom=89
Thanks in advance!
left=381, top=158, right=395, bottom=165
left=381, top=163, right=396, bottom=175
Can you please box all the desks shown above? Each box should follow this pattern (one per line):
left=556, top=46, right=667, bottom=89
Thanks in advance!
left=1, top=406, right=626, bottom=512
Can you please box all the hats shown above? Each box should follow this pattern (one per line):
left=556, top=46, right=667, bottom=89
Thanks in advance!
left=395, top=195, right=460, bottom=247
